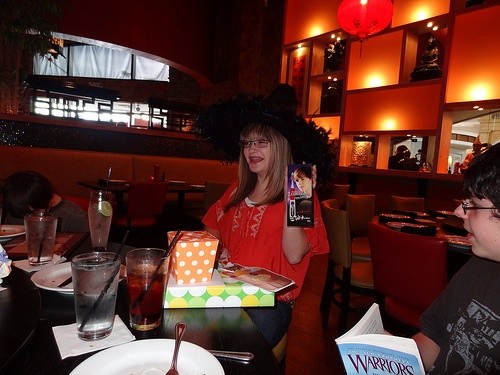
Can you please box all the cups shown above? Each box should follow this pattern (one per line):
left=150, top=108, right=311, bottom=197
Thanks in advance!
left=23, top=213, right=58, bottom=267
left=88, top=190, right=113, bottom=251
left=71, top=251, right=122, bottom=342
left=125, top=247, right=171, bottom=331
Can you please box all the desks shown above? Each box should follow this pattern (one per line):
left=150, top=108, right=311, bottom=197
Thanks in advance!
left=74, top=181, right=206, bottom=228
left=0, top=241, right=278, bottom=375
left=372, top=209, right=473, bottom=268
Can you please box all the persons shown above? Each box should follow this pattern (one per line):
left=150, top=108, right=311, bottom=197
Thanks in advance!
left=1, top=171, right=89, bottom=234
left=193, top=83, right=335, bottom=349
left=412, top=143, right=500, bottom=375
left=294, top=167, right=312, bottom=198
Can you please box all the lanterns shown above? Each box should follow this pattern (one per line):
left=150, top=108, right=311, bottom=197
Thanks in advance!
left=336, top=0, right=394, bottom=41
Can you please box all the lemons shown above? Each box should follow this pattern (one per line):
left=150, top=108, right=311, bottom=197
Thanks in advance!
left=98, top=201, right=112, bottom=217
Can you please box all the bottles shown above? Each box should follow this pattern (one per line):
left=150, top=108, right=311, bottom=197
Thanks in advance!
left=415, top=150, right=422, bottom=169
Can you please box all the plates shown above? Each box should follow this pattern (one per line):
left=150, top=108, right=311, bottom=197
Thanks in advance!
left=377, top=208, right=474, bottom=248
left=69, top=339, right=226, bottom=375
left=0, top=224, right=26, bottom=241
left=30, top=261, right=127, bottom=295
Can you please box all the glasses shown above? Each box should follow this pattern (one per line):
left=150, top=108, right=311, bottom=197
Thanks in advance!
left=462, top=201, right=500, bottom=215
left=239, top=140, right=271, bottom=148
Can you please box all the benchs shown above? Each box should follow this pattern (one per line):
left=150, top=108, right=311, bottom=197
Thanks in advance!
left=0, top=145, right=239, bottom=232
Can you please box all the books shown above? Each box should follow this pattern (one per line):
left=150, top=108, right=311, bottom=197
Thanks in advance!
left=333, top=302, right=425, bottom=375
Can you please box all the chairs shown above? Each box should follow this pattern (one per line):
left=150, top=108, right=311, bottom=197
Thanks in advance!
left=321, top=195, right=462, bottom=326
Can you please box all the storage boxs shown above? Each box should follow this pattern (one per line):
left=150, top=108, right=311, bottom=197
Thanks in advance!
left=167, top=231, right=219, bottom=285
left=162, top=270, right=273, bottom=308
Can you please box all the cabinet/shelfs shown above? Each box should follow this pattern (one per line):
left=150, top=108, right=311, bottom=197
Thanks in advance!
left=277, top=0, right=500, bottom=182
